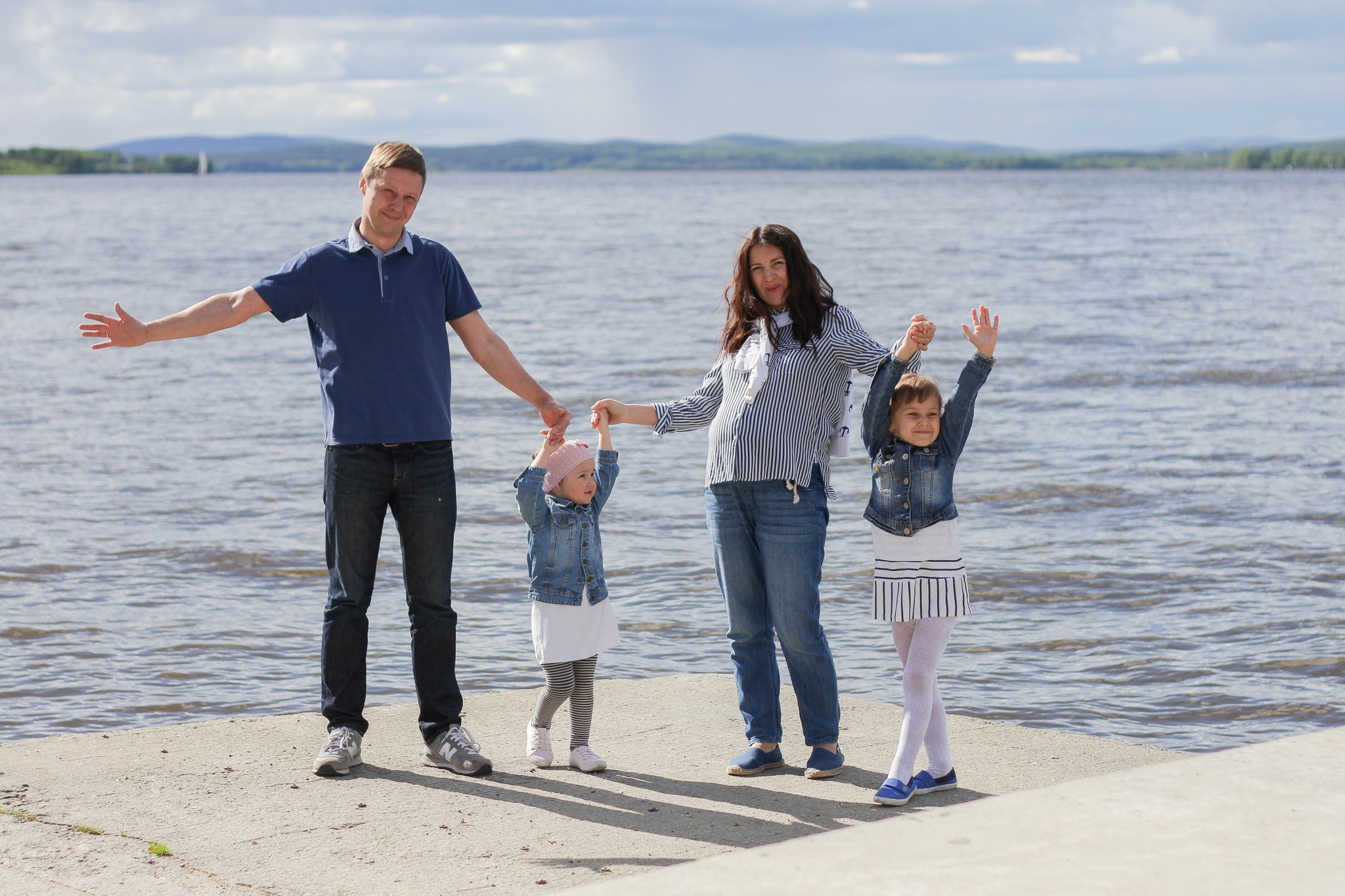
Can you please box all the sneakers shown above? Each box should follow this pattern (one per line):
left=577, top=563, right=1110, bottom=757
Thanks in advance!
left=873, top=775, right=917, bottom=805
left=313, top=726, right=363, bottom=776
left=569, top=745, right=607, bottom=772
left=913, top=766, right=957, bottom=794
left=526, top=716, right=553, bottom=768
left=421, top=723, right=492, bottom=775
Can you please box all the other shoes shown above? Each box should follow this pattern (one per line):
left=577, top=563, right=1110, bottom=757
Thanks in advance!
left=803, top=743, right=843, bottom=779
left=727, top=743, right=784, bottom=776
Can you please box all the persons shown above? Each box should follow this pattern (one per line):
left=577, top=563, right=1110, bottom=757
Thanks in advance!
left=859, top=304, right=999, bottom=803
left=80, top=143, right=569, bottom=776
left=596, top=224, right=935, bottom=778
left=514, top=406, right=621, bottom=771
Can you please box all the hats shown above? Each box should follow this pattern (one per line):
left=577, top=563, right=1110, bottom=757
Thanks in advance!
left=540, top=440, right=592, bottom=493
